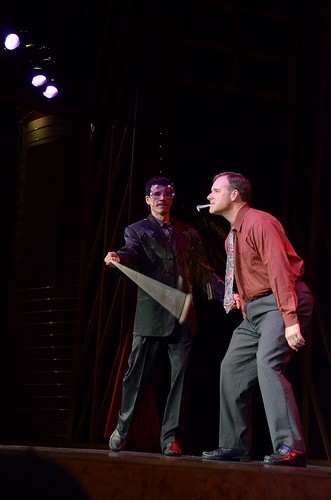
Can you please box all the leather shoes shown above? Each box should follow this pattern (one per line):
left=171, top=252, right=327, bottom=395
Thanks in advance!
left=109, top=429, right=128, bottom=452
left=264, top=445, right=307, bottom=467
left=202, top=447, right=252, bottom=462
left=164, top=441, right=183, bottom=456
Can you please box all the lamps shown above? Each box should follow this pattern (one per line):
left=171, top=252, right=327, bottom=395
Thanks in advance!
left=0, top=22, right=65, bottom=103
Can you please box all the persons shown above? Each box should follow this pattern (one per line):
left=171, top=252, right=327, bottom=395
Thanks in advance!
left=0, top=451, right=89, bottom=500
left=201, top=171, right=315, bottom=466
left=104, top=177, right=240, bottom=456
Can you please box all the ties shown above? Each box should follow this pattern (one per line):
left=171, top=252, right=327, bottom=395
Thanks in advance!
left=223, top=225, right=235, bottom=314
